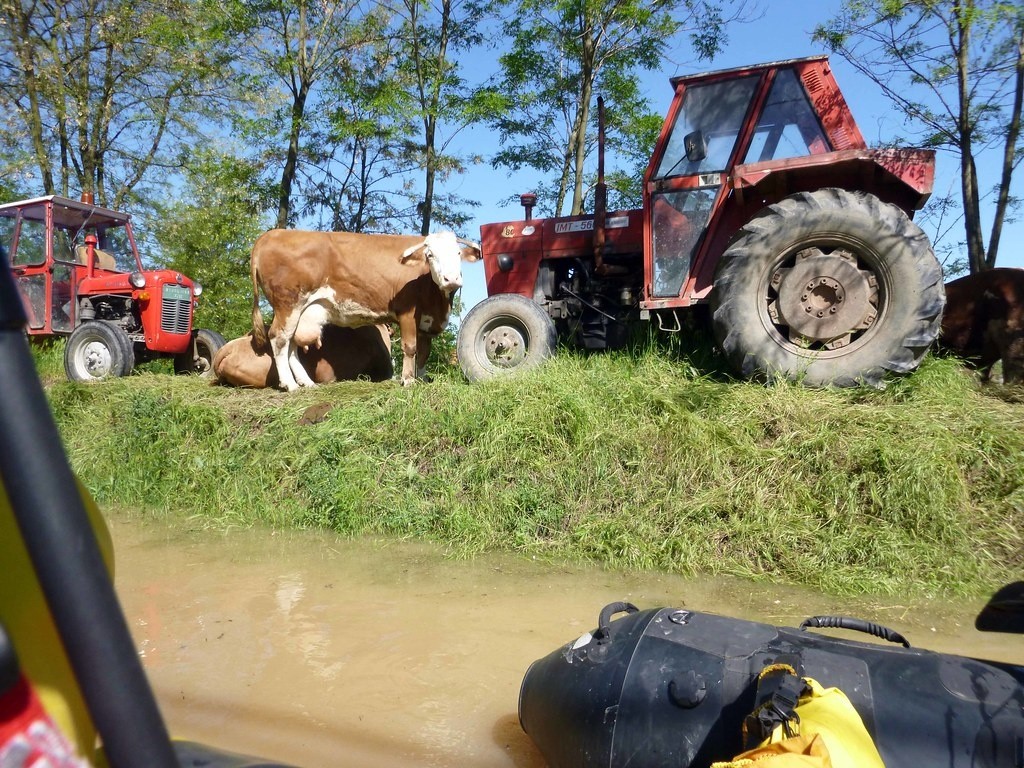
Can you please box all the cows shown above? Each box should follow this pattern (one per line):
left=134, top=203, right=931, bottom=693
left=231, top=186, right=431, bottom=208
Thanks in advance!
left=210, top=221, right=482, bottom=396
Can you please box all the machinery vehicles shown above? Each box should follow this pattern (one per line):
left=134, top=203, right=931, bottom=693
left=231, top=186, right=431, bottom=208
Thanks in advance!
left=1, top=192, right=227, bottom=388
left=457, top=55, right=948, bottom=392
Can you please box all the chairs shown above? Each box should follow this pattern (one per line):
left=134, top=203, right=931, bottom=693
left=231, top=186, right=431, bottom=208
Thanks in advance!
left=73, top=245, right=117, bottom=271
